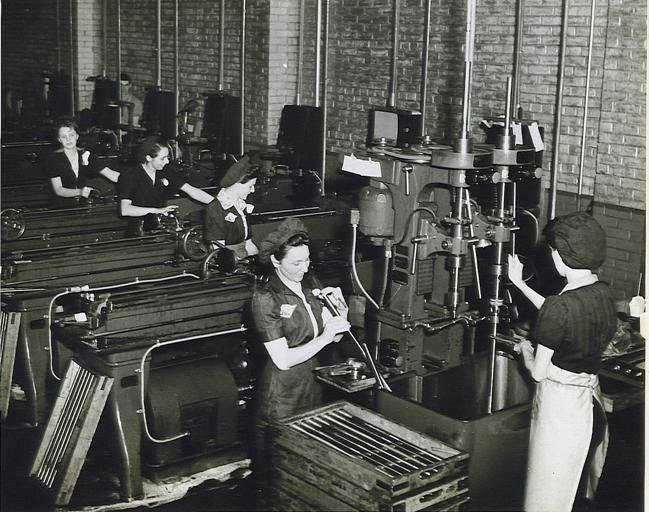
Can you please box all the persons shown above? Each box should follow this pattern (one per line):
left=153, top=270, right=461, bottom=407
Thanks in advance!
left=202, top=156, right=259, bottom=252
left=121, top=74, right=144, bottom=127
left=508, top=210, right=617, bottom=512
left=45, top=122, right=120, bottom=209
left=248, top=216, right=352, bottom=469
left=116, top=135, right=215, bottom=238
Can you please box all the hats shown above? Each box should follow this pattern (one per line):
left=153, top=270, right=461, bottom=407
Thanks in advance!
left=552, top=213, right=609, bottom=270
left=257, top=217, right=309, bottom=266
left=220, top=155, right=252, bottom=187
left=135, top=135, right=160, bottom=162
